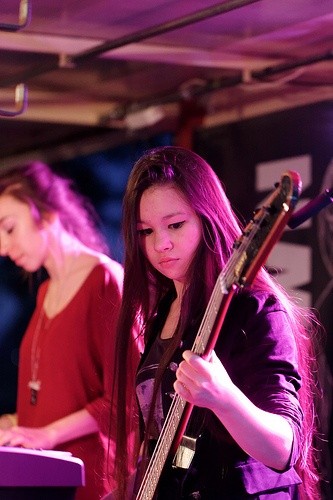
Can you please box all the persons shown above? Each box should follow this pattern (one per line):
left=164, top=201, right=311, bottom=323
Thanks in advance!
left=104, top=144, right=323, bottom=499
left=0, top=160, right=151, bottom=500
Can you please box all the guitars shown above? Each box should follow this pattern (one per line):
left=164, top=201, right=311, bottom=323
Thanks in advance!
left=134, top=168, right=303, bottom=500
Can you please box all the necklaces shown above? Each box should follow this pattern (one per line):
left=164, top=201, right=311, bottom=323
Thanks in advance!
left=28, top=246, right=89, bottom=406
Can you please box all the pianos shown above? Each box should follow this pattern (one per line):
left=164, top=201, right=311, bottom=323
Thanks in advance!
left=0, top=446, right=85, bottom=500
